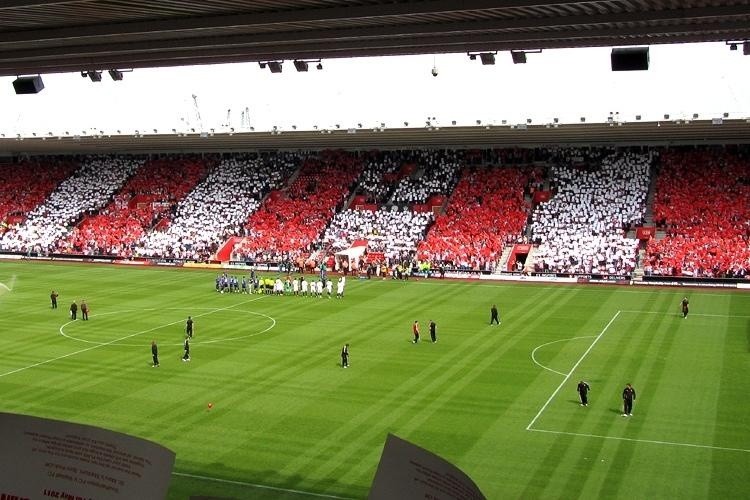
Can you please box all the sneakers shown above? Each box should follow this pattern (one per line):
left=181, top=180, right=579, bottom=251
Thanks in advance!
left=623, top=413, right=633, bottom=418
left=181, top=358, right=191, bottom=362
left=217, top=290, right=342, bottom=299
left=579, top=403, right=588, bottom=406
left=152, top=364, right=160, bottom=368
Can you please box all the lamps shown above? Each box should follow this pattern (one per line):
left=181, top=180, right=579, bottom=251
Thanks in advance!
left=153, top=125, right=298, bottom=136
left=257, top=59, right=286, bottom=73
left=313, top=116, right=440, bottom=134
left=466, top=50, right=500, bottom=68
left=608, top=43, right=654, bottom=73
left=293, top=56, right=324, bottom=77
left=452, top=117, right=586, bottom=130
left=722, top=39, right=750, bottom=55
left=106, top=67, right=135, bottom=81
left=0, top=130, right=143, bottom=142
left=10, top=72, right=47, bottom=97
left=509, top=49, right=543, bottom=67
left=607, top=111, right=730, bottom=128
left=81, top=67, right=105, bottom=86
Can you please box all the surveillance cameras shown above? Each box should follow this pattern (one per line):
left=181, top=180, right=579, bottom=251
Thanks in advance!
left=431, top=65, right=439, bottom=77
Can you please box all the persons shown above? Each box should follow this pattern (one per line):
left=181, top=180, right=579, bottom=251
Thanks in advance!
left=623, top=383, right=636, bottom=417
left=151, top=340, right=160, bottom=367
left=341, top=343, right=350, bottom=368
left=70, top=300, right=77, bottom=320
left=412, top=321, right=420, bottom=345
left=81, top=300, right=88, bottom=320
left=576, top=381, right=591, bottom=407
left=182, top=337, right=191, bottom=362
left=185, top=316, right=194, bottom=339
left=680, top=296, right=689, bottom=320
left=428, top=320, right=437, bottom=344
left=490, top=305, right=500, bottom=325
left=50, top=291, right=58, bottom=308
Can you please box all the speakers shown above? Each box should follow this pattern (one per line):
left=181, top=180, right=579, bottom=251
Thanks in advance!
left=12, top=76, right=45, bottom=94
left=611, top=47, right=650, bottom=71
